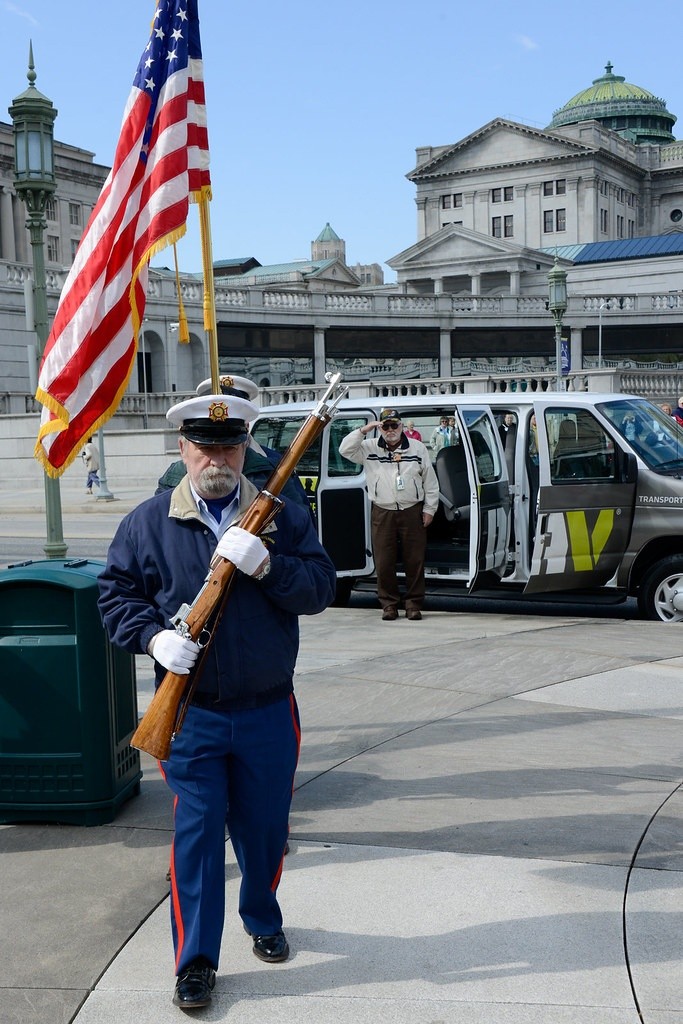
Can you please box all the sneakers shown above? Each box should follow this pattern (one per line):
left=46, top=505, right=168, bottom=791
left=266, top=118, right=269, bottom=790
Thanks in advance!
left=405, top=607, right=422, bottom=620
left=382, top=607, right=399, bottom=620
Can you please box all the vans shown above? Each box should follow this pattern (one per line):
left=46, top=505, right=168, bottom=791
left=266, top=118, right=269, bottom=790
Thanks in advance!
left=246, top=390, right=683, bottom=624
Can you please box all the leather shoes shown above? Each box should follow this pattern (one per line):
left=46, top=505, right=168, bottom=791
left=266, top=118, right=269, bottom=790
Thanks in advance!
left=172, top=958, right=216, bottom=1008
left=243, top=921, right=289, bottom=961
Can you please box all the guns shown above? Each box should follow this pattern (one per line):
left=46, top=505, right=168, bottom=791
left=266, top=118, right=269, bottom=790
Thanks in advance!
left=128, top=372, right=352, bottom=763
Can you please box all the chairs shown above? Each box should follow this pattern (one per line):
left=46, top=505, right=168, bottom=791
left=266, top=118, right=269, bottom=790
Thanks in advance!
left=436, top=444, right=470, bottom=521
left=459, top=430, right=494, bottom=479
left=553, top=419, right=593, bottom=477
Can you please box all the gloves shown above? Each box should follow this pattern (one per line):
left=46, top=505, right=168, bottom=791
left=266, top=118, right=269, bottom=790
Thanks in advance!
left=153, top=629, right=200, bottom=674
left=215, top=526, right=268, bottom=576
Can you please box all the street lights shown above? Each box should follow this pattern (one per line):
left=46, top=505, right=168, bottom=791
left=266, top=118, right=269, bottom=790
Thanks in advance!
left=547, top=243, right=568, bottom=391
left=8, top=38, right=72, bottom=558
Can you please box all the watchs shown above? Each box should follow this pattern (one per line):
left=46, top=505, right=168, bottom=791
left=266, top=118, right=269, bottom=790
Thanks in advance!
left=254, top=554, right=271, bottom=580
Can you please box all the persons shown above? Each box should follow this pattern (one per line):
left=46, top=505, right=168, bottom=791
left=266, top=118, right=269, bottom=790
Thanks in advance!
left=448, top=417, right=459, bottom=446
left=670, top=397, right=683, bottom=428
left=403, top=421, right=421, bottom=442
left=399, top=480, right=402, bottom=485
left=498, top=414, right=516, bottom=452
left=81, top=437, right=100, bottom=494
left=529, top=415, right=540, bottom=465
left=338, top=410, right=439, bottom=621
left=430, top=416, right=457, bottom=460
left=653, top=403, right=677, bottom=444
left=619, top=410, right=643, bottom=442
left=96, top=395, right=336, bottom=1009
left=154, top=375, right=318, bottom=534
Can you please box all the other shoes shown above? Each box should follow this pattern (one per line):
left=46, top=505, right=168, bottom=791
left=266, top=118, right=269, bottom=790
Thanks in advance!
left=84, top=488, right=92, bottom=494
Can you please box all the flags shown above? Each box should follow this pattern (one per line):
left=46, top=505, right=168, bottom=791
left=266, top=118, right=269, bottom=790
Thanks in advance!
left=33, top=0, right=214, bottom=478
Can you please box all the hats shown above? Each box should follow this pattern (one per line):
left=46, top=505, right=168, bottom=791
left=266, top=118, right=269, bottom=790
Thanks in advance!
left=380, top=409, right=401, bottom=422
left=166, top=394, right=260, bottom=445
left=196, top=374, right=259, bottom=402
left=678, top=397, right=683, bottom=403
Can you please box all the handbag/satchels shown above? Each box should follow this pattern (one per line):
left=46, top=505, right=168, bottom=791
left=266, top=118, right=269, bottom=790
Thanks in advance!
left=82, top=452, right=91, bottom=462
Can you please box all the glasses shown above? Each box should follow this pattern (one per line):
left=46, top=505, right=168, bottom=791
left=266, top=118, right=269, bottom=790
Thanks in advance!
left=678, top=403, right=683, bottom=404
left=441, top=421, right=447, bottom=423
left=381, top=423, right=401, bottom=431
